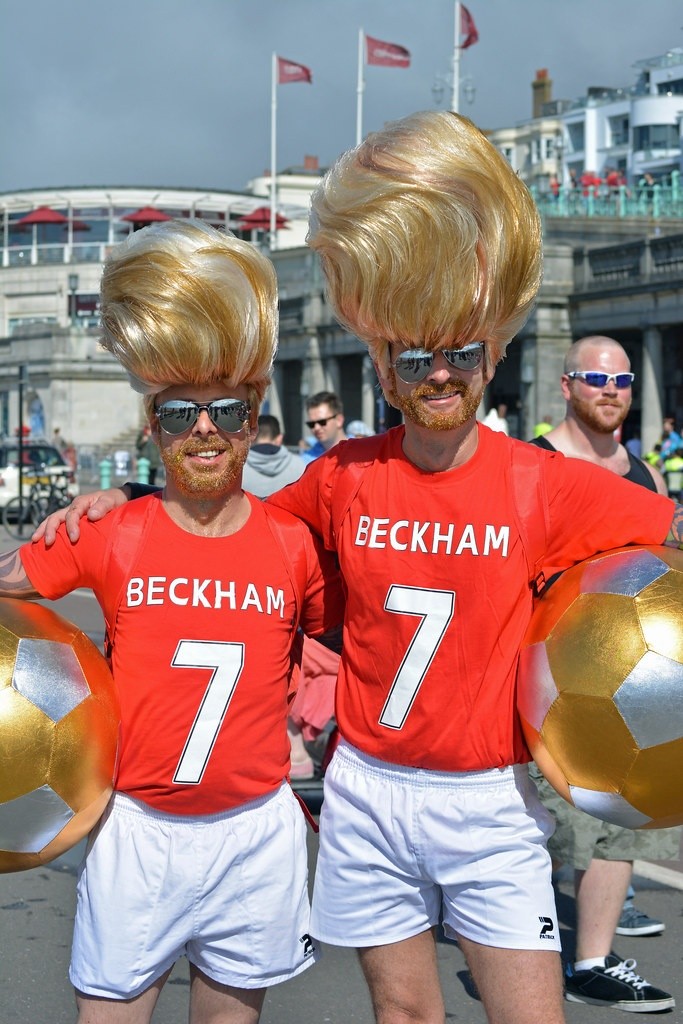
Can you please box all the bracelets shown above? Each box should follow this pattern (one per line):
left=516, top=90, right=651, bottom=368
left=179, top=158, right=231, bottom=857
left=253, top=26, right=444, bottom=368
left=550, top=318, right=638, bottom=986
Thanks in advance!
left=124, top=482, right=164, bottom=500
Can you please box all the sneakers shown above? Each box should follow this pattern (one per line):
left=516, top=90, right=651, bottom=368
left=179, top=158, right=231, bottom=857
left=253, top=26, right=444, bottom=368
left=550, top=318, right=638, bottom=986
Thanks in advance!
left=566, top=954, right=676, bottom=1013
left=616, top=906, right=666, bottom=936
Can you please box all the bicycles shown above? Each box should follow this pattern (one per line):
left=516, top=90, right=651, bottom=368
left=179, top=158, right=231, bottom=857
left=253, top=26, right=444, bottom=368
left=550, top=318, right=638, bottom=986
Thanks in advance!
left=2, top=467, right=74, bottom=540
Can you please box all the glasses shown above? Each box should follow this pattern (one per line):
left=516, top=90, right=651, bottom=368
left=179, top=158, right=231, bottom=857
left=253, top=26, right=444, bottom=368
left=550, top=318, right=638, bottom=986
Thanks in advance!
left=305, top=414, right=337, bottom=428
left=567, top=370, right=637, bottom=389
left=390, top=341, right=485, bottom=384
left=155, top=397, right=250, bottom=436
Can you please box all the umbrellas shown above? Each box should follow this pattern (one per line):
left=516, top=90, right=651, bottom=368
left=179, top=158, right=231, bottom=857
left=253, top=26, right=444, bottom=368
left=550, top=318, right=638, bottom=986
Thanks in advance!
left=239, top=207, right=290, bottom=231
left=122, top=207, right=171, bottom=221
left=17, top=206, right=68, bottom=243
left=63, top=221, right=91, bottom=232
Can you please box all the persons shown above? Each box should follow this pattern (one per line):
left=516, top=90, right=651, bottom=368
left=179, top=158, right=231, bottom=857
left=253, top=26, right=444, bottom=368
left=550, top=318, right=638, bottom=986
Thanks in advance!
left=548, top=168, right=662, bottom=217
left=30, top=111, right=676, bottom=1024
left=241, top=391, right=377, bottom=498
left=482, top=404, right=683, bottom=503
left=51, top=428, right=67, bottom=454
left=0, top=223, right=347, bottom=1024
left=525, top=334, right=678, bottom=1014
left=136, top=423, right=161, bottom=485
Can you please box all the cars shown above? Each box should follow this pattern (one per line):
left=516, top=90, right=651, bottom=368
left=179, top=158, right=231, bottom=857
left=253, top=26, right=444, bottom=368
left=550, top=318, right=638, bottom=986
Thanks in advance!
left=0, top=443, right=79, bottom=522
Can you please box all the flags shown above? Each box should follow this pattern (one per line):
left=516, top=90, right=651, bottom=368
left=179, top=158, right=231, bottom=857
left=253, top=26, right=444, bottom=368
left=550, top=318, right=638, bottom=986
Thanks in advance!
left=277, top=56, right=311, bottom=84
left=365, top=35, right=411, bottom=68
left=460, top=4, right=479, bottom=49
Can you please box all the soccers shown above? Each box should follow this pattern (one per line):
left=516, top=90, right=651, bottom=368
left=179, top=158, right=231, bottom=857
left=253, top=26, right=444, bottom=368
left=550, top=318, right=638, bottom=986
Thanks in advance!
left=514, top=542, right=683, bottom=833
left=1, top=595, right=123, bottom=874
